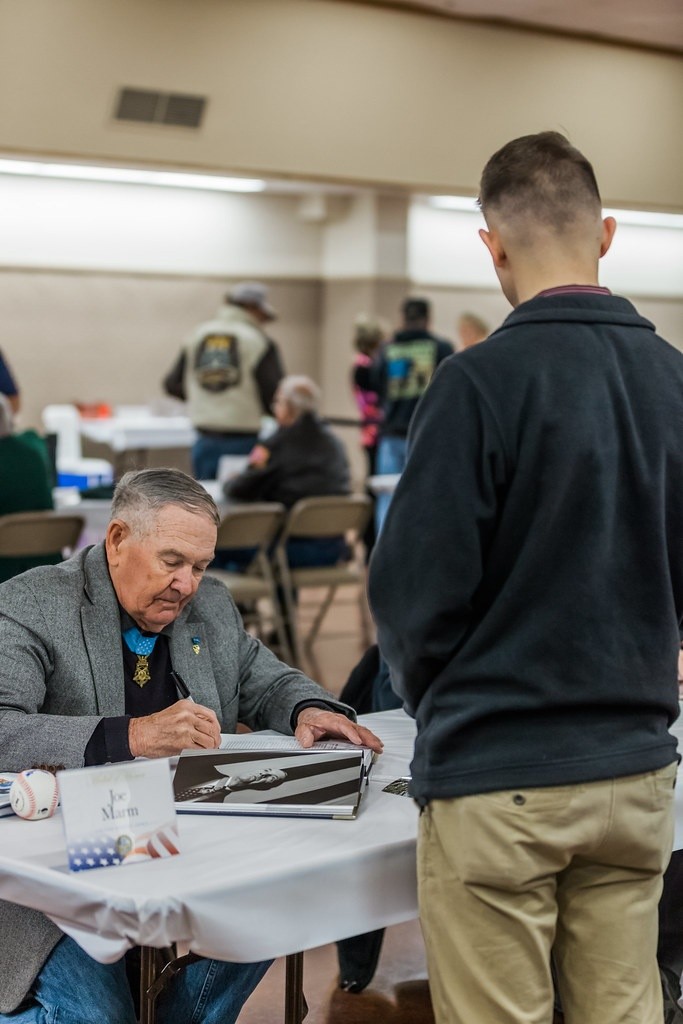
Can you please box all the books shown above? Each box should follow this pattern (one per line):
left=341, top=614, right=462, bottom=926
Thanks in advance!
left=0, top=772, right=23, bottom=818
left=171, top=733, right=379, bottom=821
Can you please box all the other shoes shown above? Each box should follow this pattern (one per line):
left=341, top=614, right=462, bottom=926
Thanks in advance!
left=267, top=625, right=298, bottom=645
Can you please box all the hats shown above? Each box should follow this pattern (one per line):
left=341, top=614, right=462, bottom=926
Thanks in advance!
left=229, top=281, right=276, bottom=320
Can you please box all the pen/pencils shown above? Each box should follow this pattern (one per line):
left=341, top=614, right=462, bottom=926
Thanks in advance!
left=165, top=668, right=224, bottom=752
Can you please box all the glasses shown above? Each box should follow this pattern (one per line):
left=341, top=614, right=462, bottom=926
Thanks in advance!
left=270, top=397, right=279, bottom=403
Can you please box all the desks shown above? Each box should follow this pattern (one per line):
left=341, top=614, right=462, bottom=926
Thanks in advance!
left=0, top=706, right=683, bottom=1024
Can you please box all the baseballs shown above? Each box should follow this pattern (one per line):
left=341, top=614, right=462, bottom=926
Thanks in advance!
left=8, top=767, right=61, bottom=821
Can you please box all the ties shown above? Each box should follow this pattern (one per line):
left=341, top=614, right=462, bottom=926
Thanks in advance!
left=175, top=785, right=222, bottom=801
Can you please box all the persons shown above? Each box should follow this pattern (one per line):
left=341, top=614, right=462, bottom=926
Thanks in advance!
left=173, top=758, right=287, bottom=804
left=160, top=280, right=283, bottom=480
left=347, top=296, right=488, bottom=567
left=0, top=468, right=386, bottom=1024
left=0, top=353, right=65, bottom=582
left=203, top=375, right=354, bottom=668
left=366, top=132, right=683, bottom=1024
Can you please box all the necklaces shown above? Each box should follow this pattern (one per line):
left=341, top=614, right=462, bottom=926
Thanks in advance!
left=122, top=628, right=158, bottom=688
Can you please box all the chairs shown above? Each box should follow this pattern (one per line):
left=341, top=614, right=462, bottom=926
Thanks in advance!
left=1, top=511, right=86, bottom=559
left=272, top=496, right=375, bottom=669
left=205, top=503, right=293, bottom=669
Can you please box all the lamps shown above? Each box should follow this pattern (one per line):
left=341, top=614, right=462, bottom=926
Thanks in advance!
left=409, top=192, right=683, bottom=229
left=0, top=153, right=360, bottom=200
